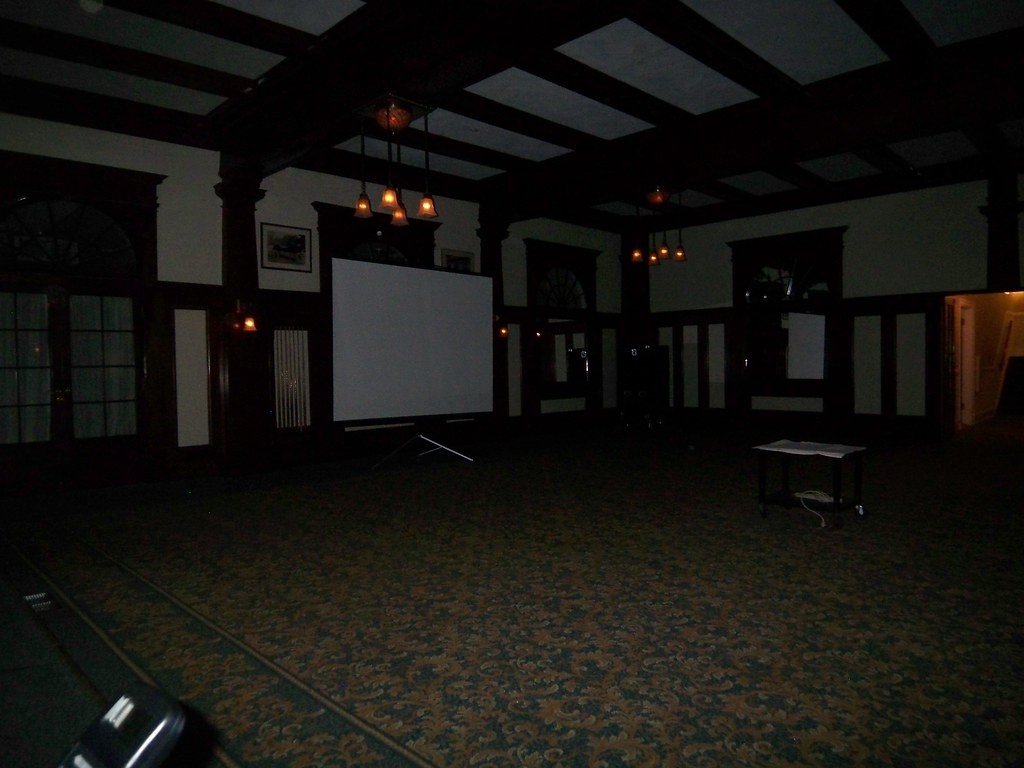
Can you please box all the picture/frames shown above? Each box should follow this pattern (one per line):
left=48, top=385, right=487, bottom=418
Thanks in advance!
left=260, top=222, right=312, bottom=273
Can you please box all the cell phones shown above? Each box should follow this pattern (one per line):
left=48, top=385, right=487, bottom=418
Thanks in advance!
left=57, top=680, right=185, bottom=768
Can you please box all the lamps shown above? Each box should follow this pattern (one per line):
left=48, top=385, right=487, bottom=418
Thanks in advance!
left=242, top=315, right=257, bottom=331
left=353, top=76, right=438, bottom=225
left=631, top=166, right=685, bottom=264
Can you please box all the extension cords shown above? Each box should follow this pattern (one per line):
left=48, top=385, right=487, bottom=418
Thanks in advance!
left=795, top=492, right=834, bottom=503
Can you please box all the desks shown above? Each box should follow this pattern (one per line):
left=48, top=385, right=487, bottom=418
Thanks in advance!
left=754, top=441, right=868, bottom=527
